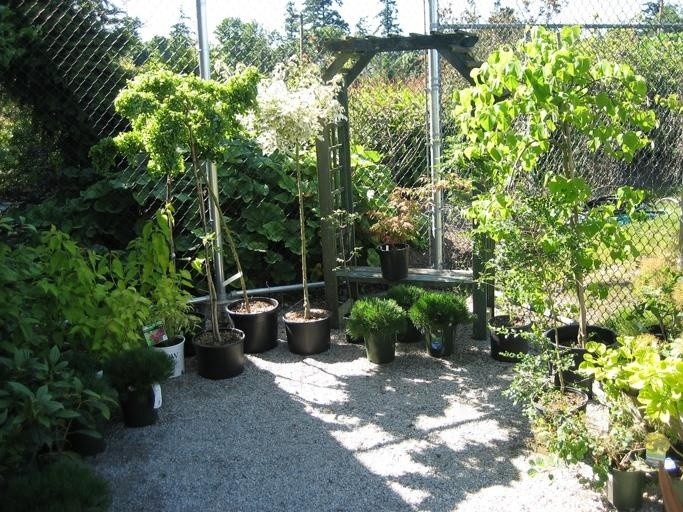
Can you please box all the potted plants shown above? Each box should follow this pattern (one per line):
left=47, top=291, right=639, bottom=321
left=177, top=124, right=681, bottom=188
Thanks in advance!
left=343, top=294, right=407, bottom=368
left=407, top=288, right=468, bottom=358
left=319, top=194, right=370, bottom=348
left=382, top=279, right=430, bottom=344
left=158, top=73, right=282, bottom=349
left=466, top=185, right=590, bottom=433
left=444, top=21, right=663, bottom=400
left=587, top=407, right=652, bottom=512
left=451, top=208, right=537, bottom=365
left=242, top=61, right=332, bottom=354
left=117, top=73, right=250, bottom=376
left=362, top=172, right=473, bottom=280
left=79, top=202, right=186, bottom=432
